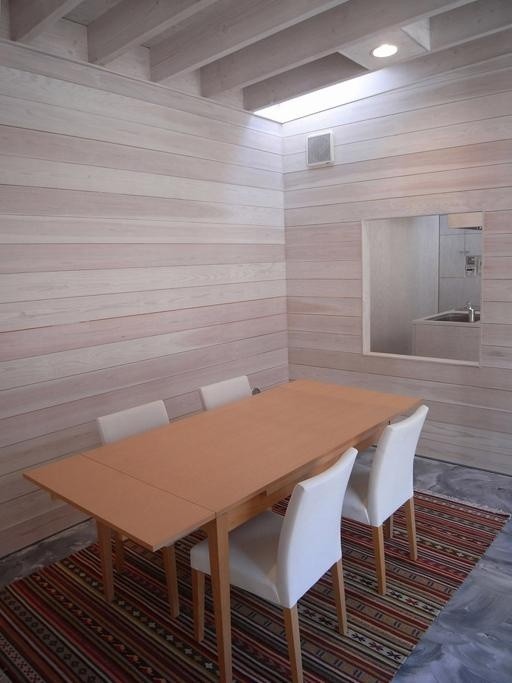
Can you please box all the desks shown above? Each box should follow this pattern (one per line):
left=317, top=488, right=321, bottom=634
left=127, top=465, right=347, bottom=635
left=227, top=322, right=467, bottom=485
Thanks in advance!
left=19, top=376, right=425, bottom=681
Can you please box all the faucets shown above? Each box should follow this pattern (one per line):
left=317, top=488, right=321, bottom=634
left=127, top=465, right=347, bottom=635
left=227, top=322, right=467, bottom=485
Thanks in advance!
left=464, top=301, right=476, bottom=324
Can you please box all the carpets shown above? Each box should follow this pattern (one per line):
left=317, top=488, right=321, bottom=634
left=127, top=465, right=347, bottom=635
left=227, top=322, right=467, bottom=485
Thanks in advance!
left=0, top=491, right=507, bottom=683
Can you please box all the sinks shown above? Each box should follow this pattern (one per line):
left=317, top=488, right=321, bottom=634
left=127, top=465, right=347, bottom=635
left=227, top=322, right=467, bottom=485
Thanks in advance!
left=424, top=313, right=481, bottom=324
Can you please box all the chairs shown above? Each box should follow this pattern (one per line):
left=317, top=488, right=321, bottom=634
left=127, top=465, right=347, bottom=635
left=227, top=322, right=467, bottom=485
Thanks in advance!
left=99, top=398, right=180, bottom=620
left=197, top=377, right=255, bottom=411
left=344, top=406, right=432, bottom=594
left=191, top=448, right=359, bottom=683
